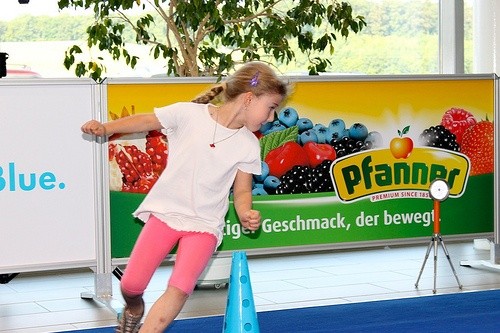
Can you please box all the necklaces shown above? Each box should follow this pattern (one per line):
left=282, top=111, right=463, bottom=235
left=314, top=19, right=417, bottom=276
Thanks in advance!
left=208, top=110, right=241, bottom=149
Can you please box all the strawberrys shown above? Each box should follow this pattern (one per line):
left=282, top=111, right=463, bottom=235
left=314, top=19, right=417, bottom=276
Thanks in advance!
left=460, top=115, right=494, bottom=176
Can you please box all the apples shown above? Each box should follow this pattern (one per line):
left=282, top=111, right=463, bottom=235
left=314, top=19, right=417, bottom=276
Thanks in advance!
left=390, top=125, right=414, bottom=159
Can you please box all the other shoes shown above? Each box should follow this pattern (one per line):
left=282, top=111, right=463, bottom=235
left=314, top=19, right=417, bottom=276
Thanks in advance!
left=119, top=307, right=143, bottom=333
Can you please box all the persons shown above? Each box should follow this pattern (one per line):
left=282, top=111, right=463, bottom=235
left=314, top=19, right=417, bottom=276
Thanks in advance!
left=81, top=61, right=293, bottom=333
